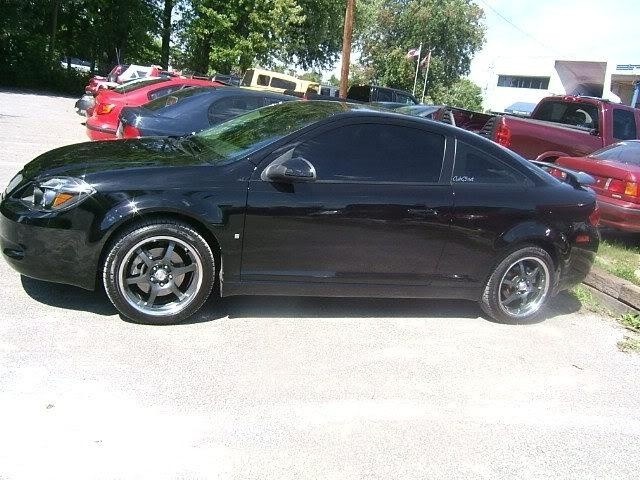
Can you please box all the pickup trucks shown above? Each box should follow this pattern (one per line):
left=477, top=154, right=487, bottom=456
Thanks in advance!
left=433, top=95, right=640, bottom=163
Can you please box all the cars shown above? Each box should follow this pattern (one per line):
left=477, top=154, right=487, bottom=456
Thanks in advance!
left=554, top=140, right=640, bottom=236
